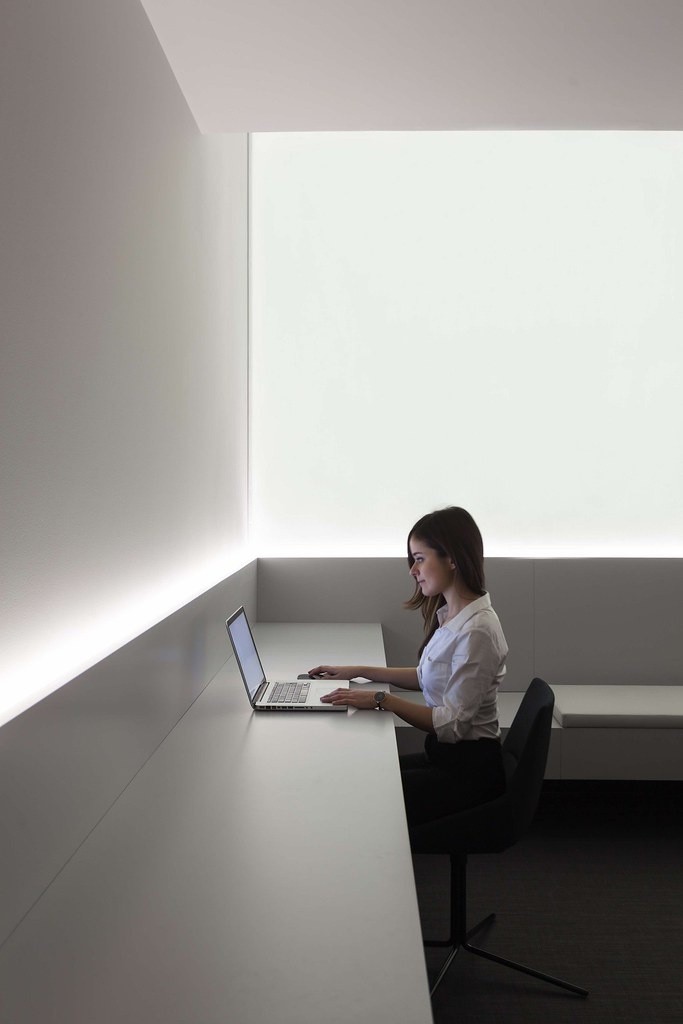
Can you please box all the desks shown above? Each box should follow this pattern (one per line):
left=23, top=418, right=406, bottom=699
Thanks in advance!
left=0, top=623, right=433, bottom=1024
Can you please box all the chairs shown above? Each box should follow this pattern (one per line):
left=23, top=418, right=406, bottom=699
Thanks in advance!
left=423, top=677, right=590, bottom=1019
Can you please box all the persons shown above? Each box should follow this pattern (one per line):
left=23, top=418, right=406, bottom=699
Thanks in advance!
left=307, top=507, right=509, bottom=827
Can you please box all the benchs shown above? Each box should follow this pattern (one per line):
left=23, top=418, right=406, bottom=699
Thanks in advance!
left=256, top=557, right=683, bottom=781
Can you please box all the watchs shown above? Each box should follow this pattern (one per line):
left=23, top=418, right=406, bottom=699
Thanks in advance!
left=373, top=691, right=385, bottom=710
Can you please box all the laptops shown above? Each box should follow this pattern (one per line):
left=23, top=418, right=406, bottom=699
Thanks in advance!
left=224, top=606, right=349, bottom=712
